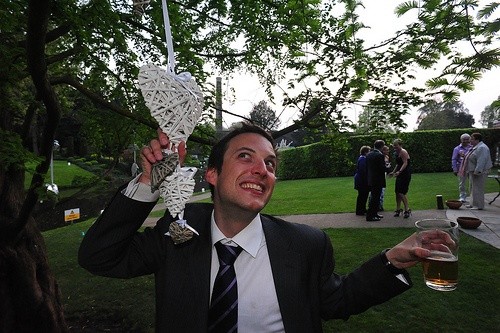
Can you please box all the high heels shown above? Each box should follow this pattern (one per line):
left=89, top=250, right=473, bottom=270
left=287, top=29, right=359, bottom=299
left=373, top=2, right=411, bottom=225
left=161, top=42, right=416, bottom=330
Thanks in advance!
left=403, top=209, right=411, bottom=218
left=394, top=208, right=403, bottom=216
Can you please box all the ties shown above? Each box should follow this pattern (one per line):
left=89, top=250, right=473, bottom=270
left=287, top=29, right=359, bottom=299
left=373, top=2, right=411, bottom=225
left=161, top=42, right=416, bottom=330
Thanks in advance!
left=207, top=241, right=245, bottom=333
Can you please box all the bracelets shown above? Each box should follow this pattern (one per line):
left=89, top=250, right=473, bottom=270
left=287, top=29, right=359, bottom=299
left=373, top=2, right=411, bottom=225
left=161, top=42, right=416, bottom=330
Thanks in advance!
left=399, top=170, right=402, bottom=173
left=380, top=248, right=407, bottom=275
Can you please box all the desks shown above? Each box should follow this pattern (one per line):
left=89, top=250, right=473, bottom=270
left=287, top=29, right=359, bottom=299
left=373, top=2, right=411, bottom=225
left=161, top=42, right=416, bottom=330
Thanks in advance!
left=488, top=174, right=500, bottom=205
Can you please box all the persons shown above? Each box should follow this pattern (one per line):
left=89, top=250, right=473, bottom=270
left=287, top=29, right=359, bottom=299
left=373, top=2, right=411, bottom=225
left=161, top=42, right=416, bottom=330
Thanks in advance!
left=452, top=133, right=493, bottom=210
left=78, top=120, right=457, bottom=333
left=354, top=138, right=411, bottom=221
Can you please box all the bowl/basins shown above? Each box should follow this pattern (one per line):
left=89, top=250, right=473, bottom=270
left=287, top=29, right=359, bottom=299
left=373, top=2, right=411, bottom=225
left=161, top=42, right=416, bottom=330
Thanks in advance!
left=446, top=199, right=462, bottom=209
left=457, top=217, right=481, bottom=229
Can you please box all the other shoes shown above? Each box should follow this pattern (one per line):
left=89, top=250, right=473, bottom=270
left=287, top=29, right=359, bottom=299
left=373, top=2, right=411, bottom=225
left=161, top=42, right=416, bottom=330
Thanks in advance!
left=376, top=214, right=384, bottom=218
left=370, top=217, right=381, bottom=221
left=465, top=204, right=475, bottom=208
left=471, top=207, right=482, bottom=210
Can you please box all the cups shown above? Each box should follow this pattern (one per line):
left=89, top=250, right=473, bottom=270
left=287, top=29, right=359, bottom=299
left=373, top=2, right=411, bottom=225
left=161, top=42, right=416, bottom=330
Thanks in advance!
left=436, top=195, right=443, bottom=210
left=416, top=220, right=458, bottom=291
